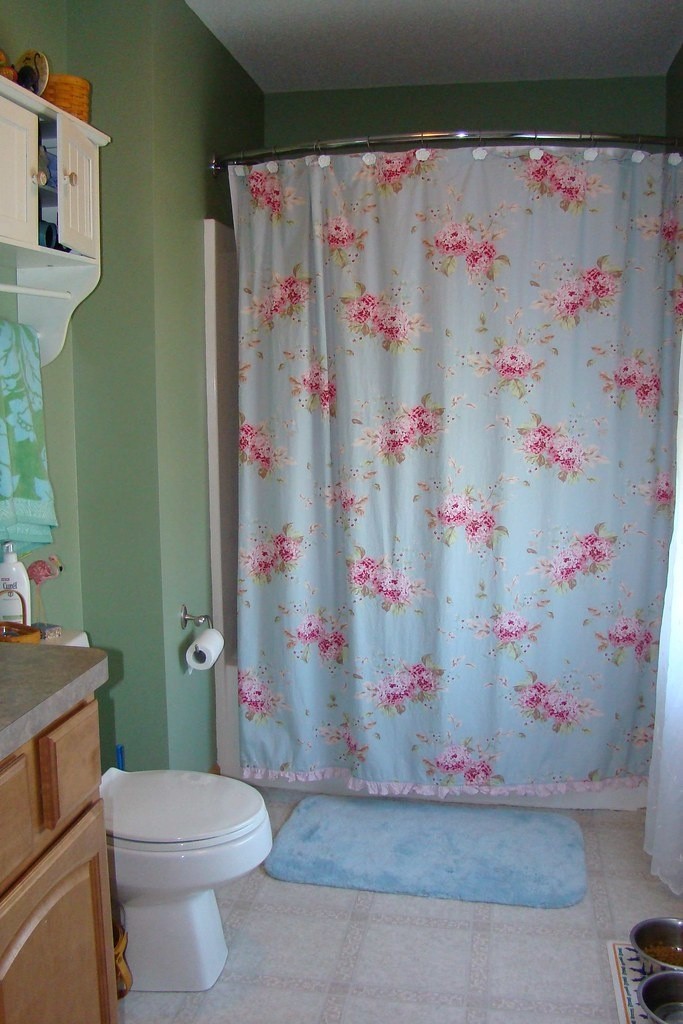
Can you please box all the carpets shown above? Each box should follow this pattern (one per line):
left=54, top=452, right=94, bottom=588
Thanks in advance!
left=263, top=794, right=588, bottom=909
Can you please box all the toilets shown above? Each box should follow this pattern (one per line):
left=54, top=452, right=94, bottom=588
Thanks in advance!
left=38, top=628, right=274, bottom=994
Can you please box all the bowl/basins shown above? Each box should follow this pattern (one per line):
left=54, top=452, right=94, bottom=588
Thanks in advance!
left=630, top=917, right=683, bottom=976
left=637, top=972, right=683, bottom=1024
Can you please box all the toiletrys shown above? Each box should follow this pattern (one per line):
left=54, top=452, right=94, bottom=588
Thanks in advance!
left=0, top=539, right=32, bottom=628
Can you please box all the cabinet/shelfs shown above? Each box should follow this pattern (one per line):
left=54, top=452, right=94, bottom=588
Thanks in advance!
left=0, top=74, right=111, bottom=369
left=0, top=690, right=118, bottom=1024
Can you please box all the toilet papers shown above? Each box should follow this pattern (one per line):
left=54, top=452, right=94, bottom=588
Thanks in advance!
left=185, top=626, right=225, bottom=677
